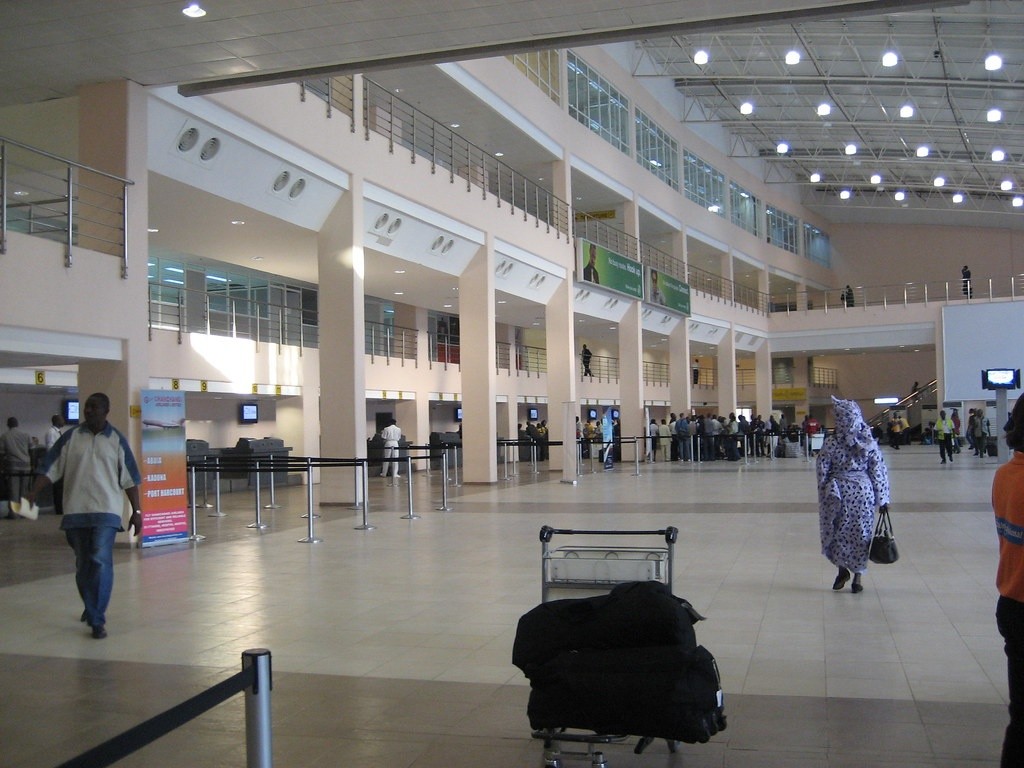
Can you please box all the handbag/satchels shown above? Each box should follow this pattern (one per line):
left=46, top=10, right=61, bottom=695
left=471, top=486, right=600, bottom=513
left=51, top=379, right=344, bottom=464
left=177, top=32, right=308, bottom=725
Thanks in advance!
left=866, top=507, right=901, bottom=563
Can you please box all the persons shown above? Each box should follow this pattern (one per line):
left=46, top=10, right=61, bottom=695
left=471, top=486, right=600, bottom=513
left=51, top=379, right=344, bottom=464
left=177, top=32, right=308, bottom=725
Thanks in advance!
left=802, top=415, right=820, bottom=435
left=0, top=417, right=36, bottom=519
left=910, top=381, right=920, bottom=406
left=576, top=416, right=621, bottom=463
left=25, top=393, right=142, bottom=640
left=961, top=266, right=972, bottom=299
left=380, top=419, right=402, bottom=479
left=951, top=408, right=961, bottom=454
left=692, top=358, right=699, bottom=384
left=583, top=243, right=600, bottom=284
left=934, top=410, right=954, bottom=464
left=966, top=409, right=991, bottom=458
left=518, top=419, right=548, bottom=461
left=649, top=412, right=786, bottom=461
left=919, top=422, right=936, bottom=445
left=846, top=284, right=854, bottom=308
left=650, top=269, right=666, bottom=306
left=890, top=412, right=911, bottom=450
left=992, top=393, right=1024, bottom=768
left=582, top=343, right=594, bottom=376
left=816, top=394, right=891, bottom=593
left=46, top=415, right=67, bottom=515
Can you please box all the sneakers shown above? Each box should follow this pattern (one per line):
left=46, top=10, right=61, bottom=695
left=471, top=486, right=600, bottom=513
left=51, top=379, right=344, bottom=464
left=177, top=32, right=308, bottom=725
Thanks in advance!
left=980, top=455, right=983, bottom=458
left=832, top=571, right=851, bottom=590
left=851, top=584, right=863, bottom=593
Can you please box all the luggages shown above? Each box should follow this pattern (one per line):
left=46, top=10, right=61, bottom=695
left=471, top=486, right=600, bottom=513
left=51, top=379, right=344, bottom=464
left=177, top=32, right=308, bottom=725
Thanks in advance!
left=987, top=436, right=998, bottom=457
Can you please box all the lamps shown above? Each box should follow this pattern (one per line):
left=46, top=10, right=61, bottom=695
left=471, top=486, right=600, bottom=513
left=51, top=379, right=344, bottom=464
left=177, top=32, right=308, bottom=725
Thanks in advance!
left=182, top=3, right=206, bottom=18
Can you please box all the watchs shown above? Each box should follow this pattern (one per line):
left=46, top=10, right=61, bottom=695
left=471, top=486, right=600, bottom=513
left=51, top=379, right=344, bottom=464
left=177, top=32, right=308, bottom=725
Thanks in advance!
left=133, top=510, right=141, bottom=514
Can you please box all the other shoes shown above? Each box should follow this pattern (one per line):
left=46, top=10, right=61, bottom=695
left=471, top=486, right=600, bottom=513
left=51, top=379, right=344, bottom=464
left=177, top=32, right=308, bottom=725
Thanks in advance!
left=948, top=455, right=953, bottom=462
left=974, top=452, right=979, bottom=455
left=380, top=473, right=386, bottom=477
left=393, top=475, right=400, bottom=478
left=940, top=460, right=946, bottom=464
left=91, top=622, right=108, bottom=639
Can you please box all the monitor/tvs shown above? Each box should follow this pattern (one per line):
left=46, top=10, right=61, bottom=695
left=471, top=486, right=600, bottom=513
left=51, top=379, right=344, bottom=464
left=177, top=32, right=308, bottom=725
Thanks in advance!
left=613, top=410, right=619, bottom=420
left=981, top=368, right=1021, bottom=390
left=588, top=410, right=597, bottom=419
left=66, top=399, right=80, bottom=425
left=456, top=408, right=462, bottom=422
left=242, top=404, right=258, bottom=424
left=529, top=409, right=538, bottom=420
